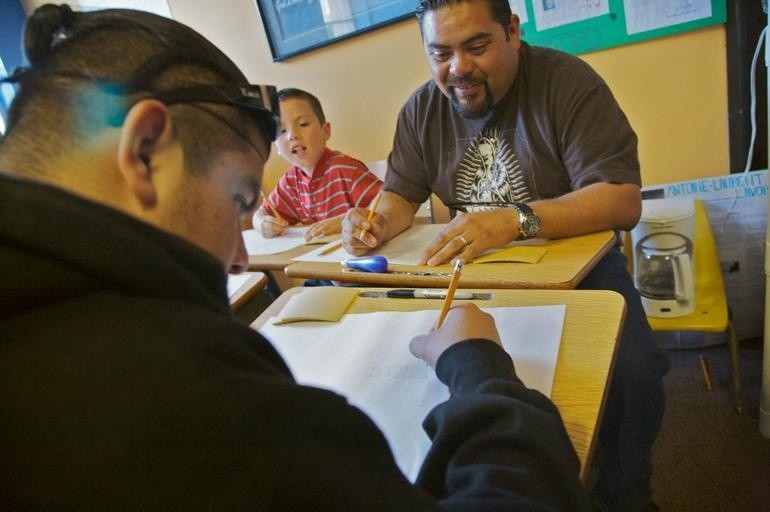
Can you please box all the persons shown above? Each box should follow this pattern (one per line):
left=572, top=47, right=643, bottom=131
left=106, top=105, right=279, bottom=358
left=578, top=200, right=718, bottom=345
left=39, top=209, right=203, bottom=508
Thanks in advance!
left=0, top=0, right=595, bottom=512
left=339, top=1, right=674, bottom=512
left=250, top=87, right=386, bottom=290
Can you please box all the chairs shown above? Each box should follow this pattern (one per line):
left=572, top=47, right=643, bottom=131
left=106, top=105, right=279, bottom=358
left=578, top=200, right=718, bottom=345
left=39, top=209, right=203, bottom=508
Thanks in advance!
left=624, top=197, right=745, bottom=414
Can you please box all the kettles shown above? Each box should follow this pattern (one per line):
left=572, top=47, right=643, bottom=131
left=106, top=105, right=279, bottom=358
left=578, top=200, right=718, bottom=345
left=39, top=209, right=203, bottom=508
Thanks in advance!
left=628, top=196, right=694, bottom=319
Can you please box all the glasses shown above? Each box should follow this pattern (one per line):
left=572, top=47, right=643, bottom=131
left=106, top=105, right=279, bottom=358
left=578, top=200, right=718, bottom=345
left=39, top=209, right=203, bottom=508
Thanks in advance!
left=107, top=82, right=284, bottom=142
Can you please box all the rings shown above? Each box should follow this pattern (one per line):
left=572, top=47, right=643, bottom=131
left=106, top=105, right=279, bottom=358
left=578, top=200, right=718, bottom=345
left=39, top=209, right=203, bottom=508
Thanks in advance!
left=457, top=235, right=469, bottom=245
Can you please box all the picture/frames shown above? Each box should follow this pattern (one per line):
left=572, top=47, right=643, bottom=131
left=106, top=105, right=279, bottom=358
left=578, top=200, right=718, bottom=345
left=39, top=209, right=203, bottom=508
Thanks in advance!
left=256, top=0, right=420, bottom=62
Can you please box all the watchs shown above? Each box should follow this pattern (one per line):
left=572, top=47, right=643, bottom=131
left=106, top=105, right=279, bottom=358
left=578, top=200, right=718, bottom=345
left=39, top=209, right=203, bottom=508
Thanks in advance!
left=505, top=202, right=543, bottom=244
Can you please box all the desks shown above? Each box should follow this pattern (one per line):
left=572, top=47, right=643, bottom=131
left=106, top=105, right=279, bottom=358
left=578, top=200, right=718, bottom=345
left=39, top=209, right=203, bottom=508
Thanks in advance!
left=284, top=223, right=618, bottom=290
left=225, top=269, right=268, bottom=313
left=249, top=285, right=632, bottom=483
left=239, top=227, right=343, bottom=269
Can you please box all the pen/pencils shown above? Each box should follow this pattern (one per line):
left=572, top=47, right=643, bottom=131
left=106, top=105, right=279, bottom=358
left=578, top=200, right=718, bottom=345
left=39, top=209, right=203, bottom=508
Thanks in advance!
left=386, top=289, right=474, bottom=299
left=360, top=192, right=382, bottom=240
left=698, top=354, right=712, bottom=392
left=426, top=258, right=465, bottom=368
left=260, top=190, right=282, bottom=222
left=318, top=242, right=342, bottom=256
